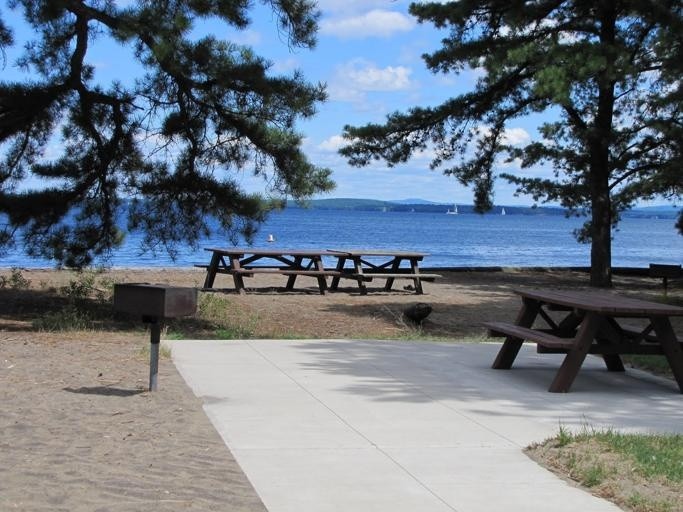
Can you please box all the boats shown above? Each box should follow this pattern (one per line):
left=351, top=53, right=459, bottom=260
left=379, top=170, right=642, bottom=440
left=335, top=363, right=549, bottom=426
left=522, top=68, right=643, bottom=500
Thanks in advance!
left=445, top=203, right=459, bottom=215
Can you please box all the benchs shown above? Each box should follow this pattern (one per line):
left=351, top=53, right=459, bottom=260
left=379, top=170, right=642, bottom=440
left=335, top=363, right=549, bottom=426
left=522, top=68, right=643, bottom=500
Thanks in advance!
left=486, top=322, right=582, bottom=393
left=196, top=264, right=441, bottom=295
left=613, top=322, right=681, bottom=387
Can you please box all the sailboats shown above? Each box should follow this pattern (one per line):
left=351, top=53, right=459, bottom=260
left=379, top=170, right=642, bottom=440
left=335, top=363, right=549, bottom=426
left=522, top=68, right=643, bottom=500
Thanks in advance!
left=500, top=207, right=505, bottom=215
left=267, top=233, right=274, bottom=242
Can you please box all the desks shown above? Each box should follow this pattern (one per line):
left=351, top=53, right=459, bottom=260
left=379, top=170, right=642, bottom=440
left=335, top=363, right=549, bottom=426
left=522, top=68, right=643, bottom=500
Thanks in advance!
left=204, top=246, right=432, bottom=268
left=513, top=283, right=681, bottom=341
